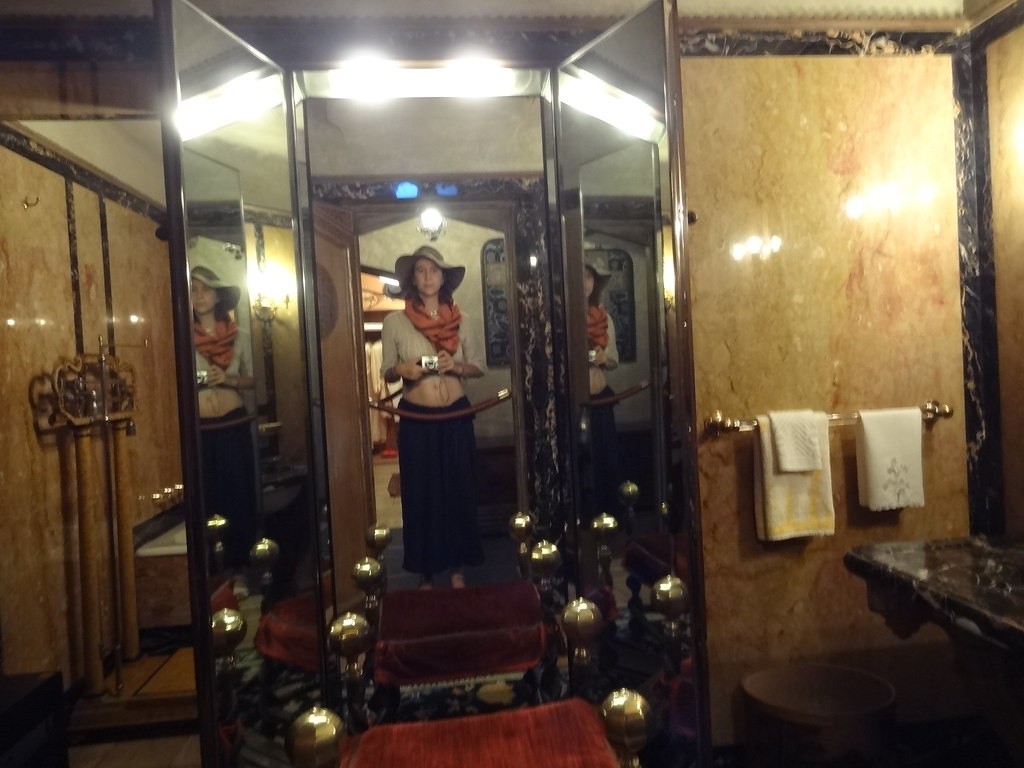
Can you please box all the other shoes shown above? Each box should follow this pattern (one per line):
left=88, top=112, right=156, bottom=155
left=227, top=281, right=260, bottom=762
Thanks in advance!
left=450, top=572, right=468, bottom=594
left=417, top=581, right=434, bottom=590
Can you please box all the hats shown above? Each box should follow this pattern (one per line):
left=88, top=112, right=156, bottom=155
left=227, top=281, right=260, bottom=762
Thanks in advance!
left=585, top=258, right=613, bottom=307
left=394, top=245, right=466, bottom=304
left=191, top=266, right=240, bottom=310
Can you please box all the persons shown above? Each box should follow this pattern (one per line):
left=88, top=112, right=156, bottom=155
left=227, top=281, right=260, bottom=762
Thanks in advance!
left=380, top=244, right=487, bottom=591
left=584, top=258, right=619, bottom=492
left=190, top=265, right=258, bottom=576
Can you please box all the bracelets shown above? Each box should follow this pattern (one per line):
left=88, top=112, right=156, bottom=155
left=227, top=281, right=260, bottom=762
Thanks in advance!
left=225, top=375, right=228, bottom=386
left=459, top=362, right=464, bottom=376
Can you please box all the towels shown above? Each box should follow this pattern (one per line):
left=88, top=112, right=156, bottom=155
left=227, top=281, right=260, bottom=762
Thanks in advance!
left=752, top=408, right=836, bottom=542
left=855, top=406, right=925, bottom=512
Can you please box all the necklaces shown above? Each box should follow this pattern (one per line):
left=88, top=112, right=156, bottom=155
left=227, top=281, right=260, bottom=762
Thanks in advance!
left=422, top=309, right=439, bottom=317
left=203, top=328, right=212, bottom=334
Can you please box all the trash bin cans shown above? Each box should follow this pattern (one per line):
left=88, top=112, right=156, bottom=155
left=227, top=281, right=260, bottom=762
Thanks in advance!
left=740, top=662, right=901, bottom=768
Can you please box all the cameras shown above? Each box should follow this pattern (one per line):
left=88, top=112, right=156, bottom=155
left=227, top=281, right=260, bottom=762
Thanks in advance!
left=421, top=355, right=439, bottom=370
left=588, top=350, right=596, bottom=361
left=197, top=371, right=208, bottom=384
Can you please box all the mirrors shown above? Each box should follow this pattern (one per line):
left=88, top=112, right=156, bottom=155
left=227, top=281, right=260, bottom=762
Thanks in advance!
left=157, top=0, right=718, bottom=768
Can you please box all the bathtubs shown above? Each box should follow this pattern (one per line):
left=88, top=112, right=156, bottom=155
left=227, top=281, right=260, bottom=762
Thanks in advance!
left=134, top=521, right=191, bottom=627
